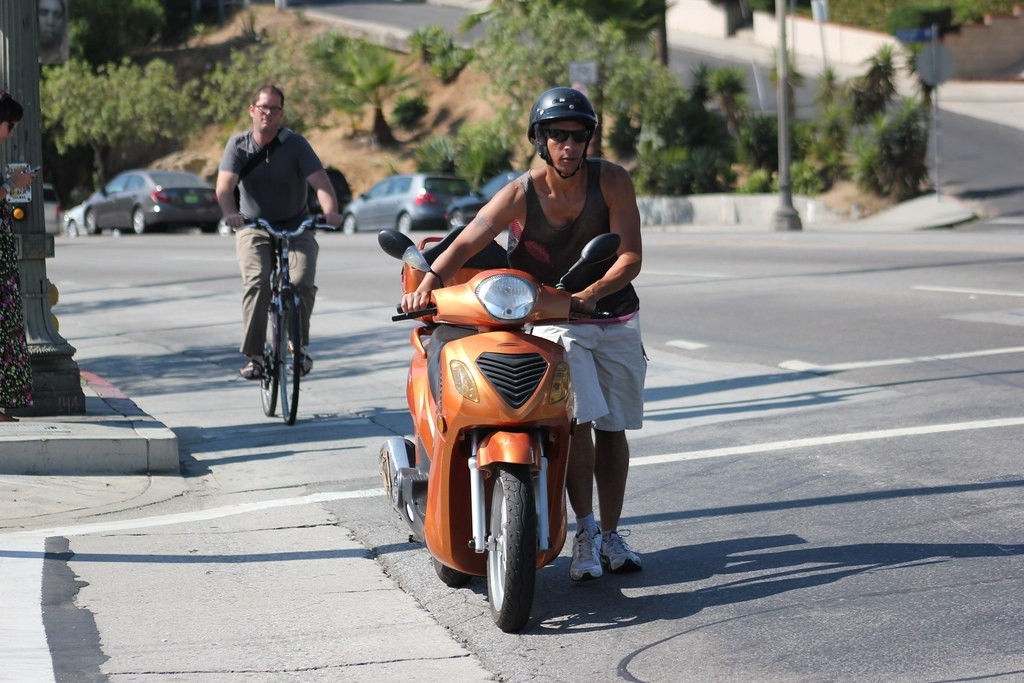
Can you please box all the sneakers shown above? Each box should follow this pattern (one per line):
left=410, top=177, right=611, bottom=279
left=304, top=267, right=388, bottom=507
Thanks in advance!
left=599, top=532, right=641, bottom=574
left=569, top=525, right=604, bottom=582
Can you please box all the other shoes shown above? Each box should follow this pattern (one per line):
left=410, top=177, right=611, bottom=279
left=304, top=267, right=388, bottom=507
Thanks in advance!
left=0, top=411, right=20, bottom=422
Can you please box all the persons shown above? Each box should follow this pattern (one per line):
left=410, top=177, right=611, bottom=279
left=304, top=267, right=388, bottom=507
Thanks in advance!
left=401, top=87, right=643, bottom=578
left=216, top=84, right=345, bottom=380
left=0, top=92, right=48, bottom=421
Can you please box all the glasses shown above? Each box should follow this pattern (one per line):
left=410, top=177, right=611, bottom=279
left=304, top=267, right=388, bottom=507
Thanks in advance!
left=543, top=129, right=590, bottom=143
left=255, top=105, right=282, bottom=115
left=6, top=121, right=17, bottom=133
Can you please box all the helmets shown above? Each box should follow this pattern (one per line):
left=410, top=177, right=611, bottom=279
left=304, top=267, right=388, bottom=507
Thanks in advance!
left=528, top=87, right=598, bottom=147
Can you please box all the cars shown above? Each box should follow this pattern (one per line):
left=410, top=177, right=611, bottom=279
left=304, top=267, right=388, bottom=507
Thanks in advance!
left=42, top=165, right=225, bottom=236
left=346, top=169, right=470, bottom=231
left=447, top=171, right=525, bottom=229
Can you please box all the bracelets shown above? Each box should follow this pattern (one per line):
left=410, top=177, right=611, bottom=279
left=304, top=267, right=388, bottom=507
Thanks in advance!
left=3, top=177, right=15, bottom=194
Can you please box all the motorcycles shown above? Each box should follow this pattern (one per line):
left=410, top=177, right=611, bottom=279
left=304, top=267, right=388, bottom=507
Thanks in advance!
left=377, top=226, right=630, bottom=630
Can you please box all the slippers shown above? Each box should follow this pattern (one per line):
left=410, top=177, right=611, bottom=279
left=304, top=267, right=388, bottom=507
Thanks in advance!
left=291, top=352, right=313, bottom=377
left=243, top=360, right=268, bottom=380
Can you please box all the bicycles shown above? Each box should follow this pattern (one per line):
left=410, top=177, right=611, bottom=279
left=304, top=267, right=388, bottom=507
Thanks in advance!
left=224, top=212, right=326, bottom=426
